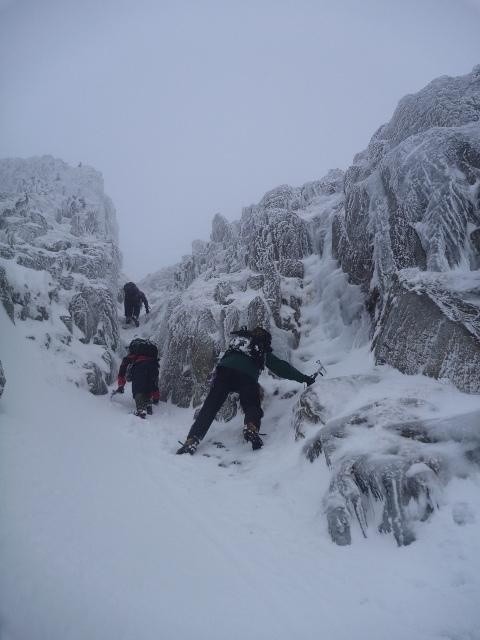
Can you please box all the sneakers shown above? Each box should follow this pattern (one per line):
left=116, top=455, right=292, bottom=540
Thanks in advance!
left=131, top=315, right=140, bottom=327
left=124, top=317, right=132, bottom=325
left=243, top=423, right=264, bottom=450
left=176, top=439, right=197, bottom=455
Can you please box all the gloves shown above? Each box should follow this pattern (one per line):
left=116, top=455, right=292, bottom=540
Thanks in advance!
left=145, top=306, right=150, bottom=313
left=153, top=398, right=160, bottom=405
left=116, top=386, right=126, bottom=393
left=303, top=374, right=316, bottom=387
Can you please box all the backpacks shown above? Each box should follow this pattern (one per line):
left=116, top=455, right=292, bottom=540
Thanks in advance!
left=124, top=282, right=139, bottom=296
left=229, top=324, right=266, bottom=358
left=129, top=338, right=159, bottom=359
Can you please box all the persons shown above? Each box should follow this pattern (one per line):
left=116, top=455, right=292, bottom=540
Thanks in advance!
left=171, top=324, right=318, bottom=455
left=117, top=281, right=149, bottom=332
left=108, top=337, right=159, bottom=418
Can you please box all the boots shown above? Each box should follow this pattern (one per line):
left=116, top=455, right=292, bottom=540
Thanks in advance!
left=145, top=400, right=153, bottom=415
left=136, top=399, right=146, bottom=417
